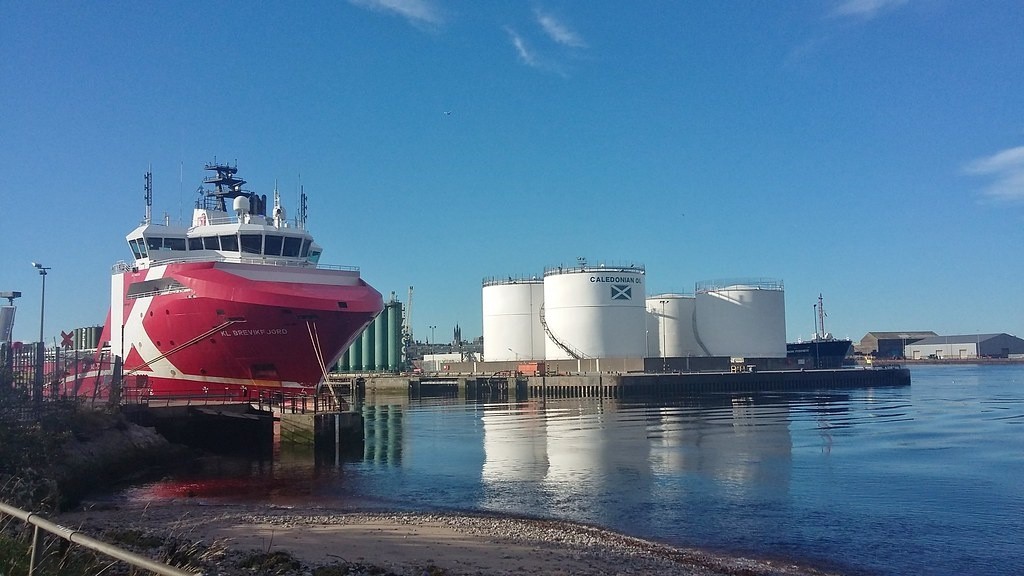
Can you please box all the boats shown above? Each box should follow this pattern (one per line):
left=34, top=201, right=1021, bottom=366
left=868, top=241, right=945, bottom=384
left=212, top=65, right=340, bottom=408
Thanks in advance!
left=1, top=154, right=386, bottom=407
